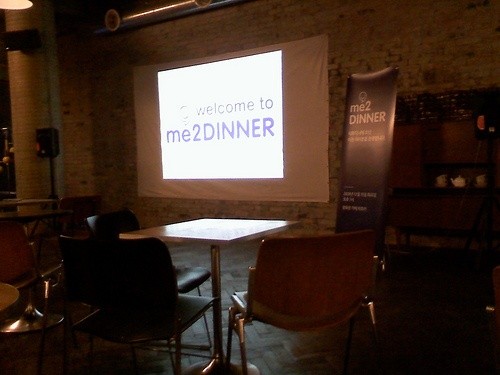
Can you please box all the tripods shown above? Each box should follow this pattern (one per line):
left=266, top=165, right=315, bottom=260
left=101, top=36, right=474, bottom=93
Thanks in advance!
left=28, top=157, right=60, bottom=241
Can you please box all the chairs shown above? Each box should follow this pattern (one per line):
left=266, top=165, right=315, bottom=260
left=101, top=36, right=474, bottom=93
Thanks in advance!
left=0, top=196, right=385, bottom=375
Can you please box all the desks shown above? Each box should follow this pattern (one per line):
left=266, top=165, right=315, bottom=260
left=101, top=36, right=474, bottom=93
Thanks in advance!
left=119, top=217, right=302, bottom=375
left=0, top=198, right=56, bottom=241
left=0, top=210, right=72, bottom=333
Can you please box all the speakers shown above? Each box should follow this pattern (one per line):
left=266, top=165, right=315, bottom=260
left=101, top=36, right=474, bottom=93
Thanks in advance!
left=1, top=29, right=42, bottom=51
left=36, top=127, right=59, bottom=157
